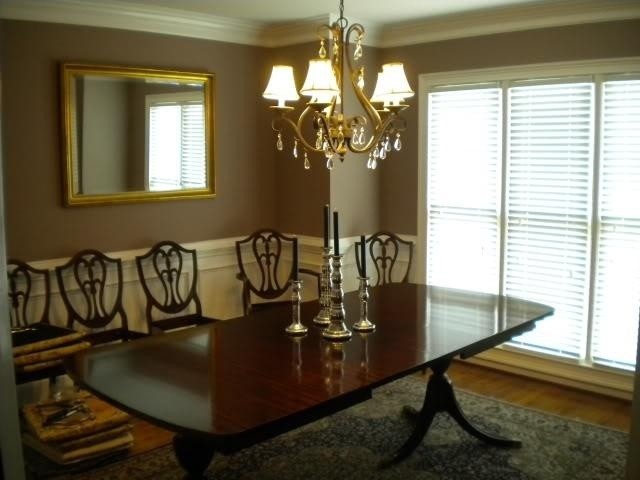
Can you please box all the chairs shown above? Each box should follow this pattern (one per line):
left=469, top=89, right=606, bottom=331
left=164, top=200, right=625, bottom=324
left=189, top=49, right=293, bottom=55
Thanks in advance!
left=135, top=241, right=223, bottom=337
left=235, top=227, right=322, bottom=315
left=7, top=259, right=88, bottom=393
left=55, top=250, right=150, bottom=355
left=354, top=231, right=415, bottom=288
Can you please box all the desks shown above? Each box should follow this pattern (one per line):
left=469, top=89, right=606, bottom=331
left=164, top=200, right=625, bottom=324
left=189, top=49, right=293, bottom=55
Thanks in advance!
left=62, top=278, right=554, bottom=478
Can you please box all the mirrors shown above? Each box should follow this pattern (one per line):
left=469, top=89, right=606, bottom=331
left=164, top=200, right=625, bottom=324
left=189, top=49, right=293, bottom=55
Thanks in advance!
left=59, top=59, right=218, bottom=208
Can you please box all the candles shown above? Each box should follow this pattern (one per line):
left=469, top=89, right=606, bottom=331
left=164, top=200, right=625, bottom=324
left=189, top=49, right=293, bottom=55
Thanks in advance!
left=292, top=238, right=299, bottom=279
left=323, top=205, right=332, bottom=246
left=333, top=211, right=340, bottom=257
left=361, top=234, right=367, bottom=277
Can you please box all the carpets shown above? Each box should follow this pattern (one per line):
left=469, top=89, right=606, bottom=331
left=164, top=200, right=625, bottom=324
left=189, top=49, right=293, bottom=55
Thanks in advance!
left=52, top=375, right=631, bottom=480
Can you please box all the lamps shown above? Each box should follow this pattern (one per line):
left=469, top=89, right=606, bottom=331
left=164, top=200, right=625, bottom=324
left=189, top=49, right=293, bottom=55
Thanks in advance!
left=262, top=0, right=417, bottom=170
